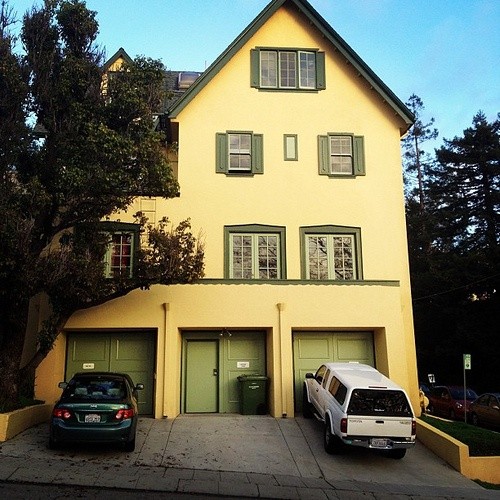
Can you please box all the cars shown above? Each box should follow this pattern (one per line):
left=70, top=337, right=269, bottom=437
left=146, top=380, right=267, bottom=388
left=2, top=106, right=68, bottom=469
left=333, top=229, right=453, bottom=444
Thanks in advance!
left=425, top=386, right=478, bottom=422
left=469, top=393, right=500, bottom=430
left=49, top=372, right=144, bottom=452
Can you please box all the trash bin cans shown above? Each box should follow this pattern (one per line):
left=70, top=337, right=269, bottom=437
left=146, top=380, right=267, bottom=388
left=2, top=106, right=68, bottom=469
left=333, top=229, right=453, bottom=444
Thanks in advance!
left=237, top=374, right=269, bottom=415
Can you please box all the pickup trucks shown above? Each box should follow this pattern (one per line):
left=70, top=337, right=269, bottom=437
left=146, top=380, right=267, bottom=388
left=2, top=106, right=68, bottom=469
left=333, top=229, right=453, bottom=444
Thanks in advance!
left=302, top=363, right=418, bottom=461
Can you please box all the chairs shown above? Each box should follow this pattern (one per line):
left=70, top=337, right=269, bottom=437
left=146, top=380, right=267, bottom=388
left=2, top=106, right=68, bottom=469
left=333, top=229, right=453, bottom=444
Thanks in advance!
left=106, top=388, right=121, bottom=396
left=75, top=388, right=88, bottom=395
